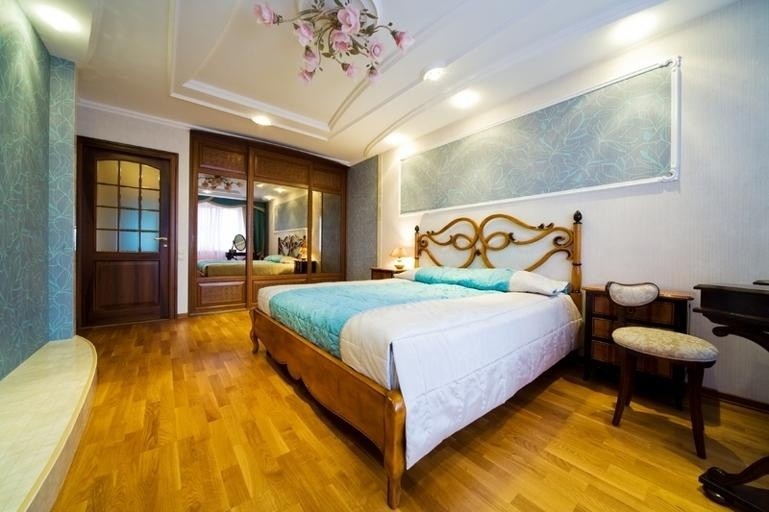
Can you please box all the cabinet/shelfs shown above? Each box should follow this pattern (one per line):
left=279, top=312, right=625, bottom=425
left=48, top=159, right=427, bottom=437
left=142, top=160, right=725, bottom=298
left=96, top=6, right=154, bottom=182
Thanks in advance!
left=189, top=128, right=350, bottom=316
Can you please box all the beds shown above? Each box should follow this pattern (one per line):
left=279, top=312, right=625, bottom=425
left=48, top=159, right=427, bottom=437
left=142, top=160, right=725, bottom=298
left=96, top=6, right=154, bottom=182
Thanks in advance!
left=248, top=210, right=585, bottom=509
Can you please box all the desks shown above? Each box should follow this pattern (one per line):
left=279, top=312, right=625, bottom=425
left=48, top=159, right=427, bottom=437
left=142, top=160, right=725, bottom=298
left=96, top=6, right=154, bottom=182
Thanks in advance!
left=692, top=281, right=769, bottom=512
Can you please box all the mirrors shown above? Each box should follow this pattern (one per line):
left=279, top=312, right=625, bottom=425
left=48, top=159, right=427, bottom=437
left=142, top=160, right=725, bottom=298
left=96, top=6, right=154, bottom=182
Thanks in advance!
left=197, top=172, right=342, bottom=280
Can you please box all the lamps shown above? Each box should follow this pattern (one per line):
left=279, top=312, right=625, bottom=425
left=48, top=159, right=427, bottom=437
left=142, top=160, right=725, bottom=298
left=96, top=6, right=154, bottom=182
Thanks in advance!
left=254, top=0, right=415, bottom=87
left=389, top=248, right=409, bottom=269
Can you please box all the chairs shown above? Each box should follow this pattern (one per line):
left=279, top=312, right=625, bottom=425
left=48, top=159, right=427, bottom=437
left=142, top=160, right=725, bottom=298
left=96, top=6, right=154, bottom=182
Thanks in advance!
left=604, top=281, right=719, bottom=459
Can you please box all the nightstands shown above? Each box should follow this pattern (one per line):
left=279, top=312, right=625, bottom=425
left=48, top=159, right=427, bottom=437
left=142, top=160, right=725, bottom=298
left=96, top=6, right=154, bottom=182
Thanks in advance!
left=368, top=266, right=407, bottom=280
left=581, top=284, right=695, bottom=411
